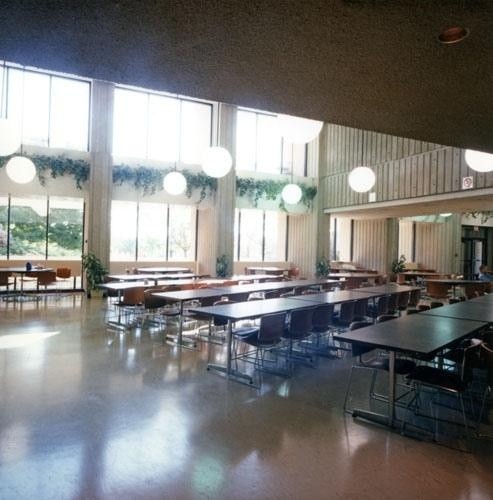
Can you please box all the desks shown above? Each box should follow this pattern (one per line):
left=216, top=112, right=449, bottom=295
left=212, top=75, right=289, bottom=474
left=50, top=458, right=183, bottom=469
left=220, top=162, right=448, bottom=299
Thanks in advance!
left=327, top=257, right=491, bottom=296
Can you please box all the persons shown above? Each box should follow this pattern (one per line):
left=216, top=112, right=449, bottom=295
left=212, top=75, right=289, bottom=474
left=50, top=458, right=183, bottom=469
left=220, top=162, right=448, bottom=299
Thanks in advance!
left=478, top=264, right=493, bottom=283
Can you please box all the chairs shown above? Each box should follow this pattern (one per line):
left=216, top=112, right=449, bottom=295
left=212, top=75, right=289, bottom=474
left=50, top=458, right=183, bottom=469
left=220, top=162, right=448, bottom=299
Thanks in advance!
left=330, top=290, right=492, bottom=453
left=95, top=267, right=423, bottom=393
left=0, top=263, right=78, bottom=304
